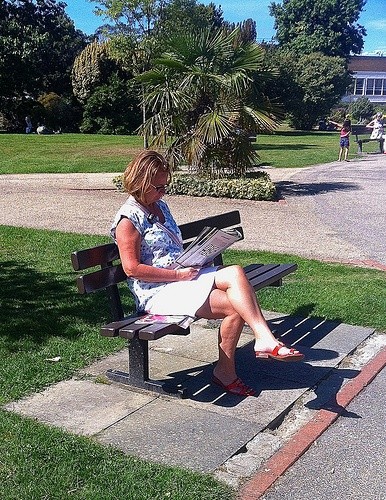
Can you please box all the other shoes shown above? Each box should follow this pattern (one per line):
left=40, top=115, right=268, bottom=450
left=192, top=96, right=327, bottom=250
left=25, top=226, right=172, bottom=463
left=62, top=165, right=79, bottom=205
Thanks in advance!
left=337, top=159, right=341, bottom=162
left=344, top=159, right=350, bottom=162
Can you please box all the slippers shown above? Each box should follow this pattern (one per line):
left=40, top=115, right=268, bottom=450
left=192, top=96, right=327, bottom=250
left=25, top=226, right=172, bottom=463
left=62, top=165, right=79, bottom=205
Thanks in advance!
left=255, top=343, right=305, bottom=362
left=211, top=378, right=256, bottom=397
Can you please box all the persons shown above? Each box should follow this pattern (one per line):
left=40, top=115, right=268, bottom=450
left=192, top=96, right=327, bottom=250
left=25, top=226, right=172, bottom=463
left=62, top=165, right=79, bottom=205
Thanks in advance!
left=110, top=150, right=305, bottom=396
left=366, top=113, right=386, bottom=155
left=330, top=119, right=352, bottom=162
left=25, top=114, right=61, bottom=135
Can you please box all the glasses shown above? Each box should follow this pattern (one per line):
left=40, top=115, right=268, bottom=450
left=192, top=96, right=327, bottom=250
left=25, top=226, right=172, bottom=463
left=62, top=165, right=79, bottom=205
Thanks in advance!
left=150, top=183, right=170, bottom=194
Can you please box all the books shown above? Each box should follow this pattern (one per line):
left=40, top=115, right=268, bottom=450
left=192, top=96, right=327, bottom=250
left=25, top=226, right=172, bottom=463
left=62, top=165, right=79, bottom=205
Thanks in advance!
left=175, top=226, right=243, bottom=269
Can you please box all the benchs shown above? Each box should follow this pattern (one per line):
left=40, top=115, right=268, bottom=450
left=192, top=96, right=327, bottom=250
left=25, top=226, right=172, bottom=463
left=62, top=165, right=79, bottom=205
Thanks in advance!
left=351, top=125, right=386, bottom=154
left=70, top=211, right=297, bottom=393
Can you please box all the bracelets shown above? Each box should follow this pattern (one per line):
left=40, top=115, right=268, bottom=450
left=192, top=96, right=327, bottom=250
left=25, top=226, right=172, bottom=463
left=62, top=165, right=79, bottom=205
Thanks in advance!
left=175, top=270, right=178, bottom=282
